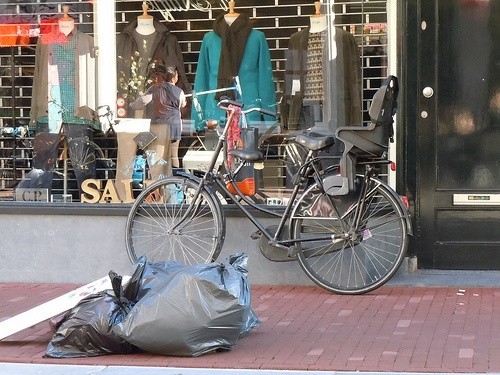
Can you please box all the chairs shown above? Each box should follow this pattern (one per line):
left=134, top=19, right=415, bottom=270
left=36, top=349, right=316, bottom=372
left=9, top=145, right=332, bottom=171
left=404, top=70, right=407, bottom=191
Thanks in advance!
left=321, top=74, right=398, bottom=196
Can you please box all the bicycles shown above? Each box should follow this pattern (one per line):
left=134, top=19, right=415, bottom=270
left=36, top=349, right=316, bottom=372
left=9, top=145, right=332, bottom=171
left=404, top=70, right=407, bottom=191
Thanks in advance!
left=0, top=98, right=117, bottom=191
left=124, top=100, right=415, bottom=296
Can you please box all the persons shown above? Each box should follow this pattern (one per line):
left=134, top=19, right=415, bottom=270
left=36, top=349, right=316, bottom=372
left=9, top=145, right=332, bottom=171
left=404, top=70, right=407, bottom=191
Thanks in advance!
left=129, top=67, right=186, bottom=167
left=116, top=15, right=192, bottom=203
left=29, top=16, right=94, bottom=200
left=284, top=13, right=361, bottom=190
left=18, top=149, right=53, bottom=188
left=191, top=12, right=277, bottom=189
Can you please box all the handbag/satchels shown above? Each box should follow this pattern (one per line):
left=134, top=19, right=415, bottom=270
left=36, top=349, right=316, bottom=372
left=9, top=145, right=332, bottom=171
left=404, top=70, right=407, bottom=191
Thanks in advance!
left=225, top=113, right=259, bottom=195
